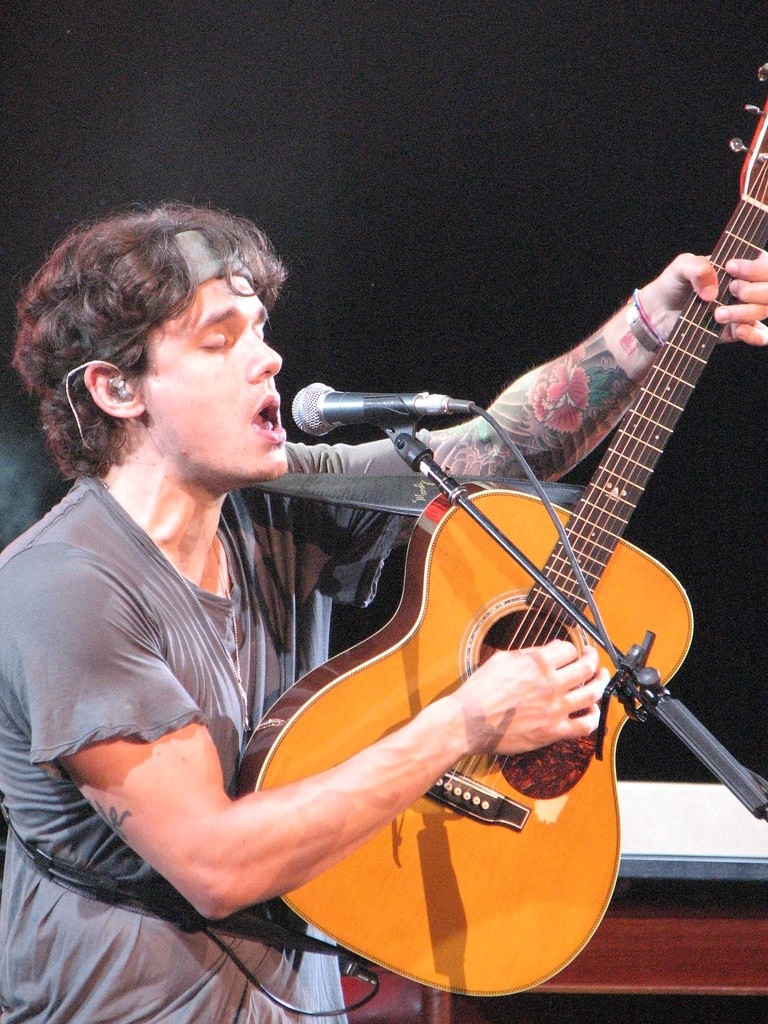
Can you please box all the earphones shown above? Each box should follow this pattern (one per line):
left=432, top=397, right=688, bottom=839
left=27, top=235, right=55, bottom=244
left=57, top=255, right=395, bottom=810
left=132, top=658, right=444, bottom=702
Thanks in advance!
left=108, top=376, right=133, bottom=404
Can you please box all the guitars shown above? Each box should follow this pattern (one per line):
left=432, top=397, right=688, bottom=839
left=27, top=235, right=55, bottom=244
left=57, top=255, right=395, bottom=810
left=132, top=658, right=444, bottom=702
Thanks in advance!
left=228, top=64, right=767, bottom=999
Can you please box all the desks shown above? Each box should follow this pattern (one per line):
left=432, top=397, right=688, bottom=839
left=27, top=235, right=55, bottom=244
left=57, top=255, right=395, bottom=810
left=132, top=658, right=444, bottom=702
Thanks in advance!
left=421, top=783, right=768, bottom=1024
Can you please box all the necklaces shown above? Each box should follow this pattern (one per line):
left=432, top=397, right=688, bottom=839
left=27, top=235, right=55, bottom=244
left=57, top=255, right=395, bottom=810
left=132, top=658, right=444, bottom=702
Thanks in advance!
left=98, top=468, right=251, bottom=733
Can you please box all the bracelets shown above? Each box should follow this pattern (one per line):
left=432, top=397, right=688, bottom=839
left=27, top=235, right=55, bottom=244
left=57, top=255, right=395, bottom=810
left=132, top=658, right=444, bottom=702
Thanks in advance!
left=625, top=288, right=666, bottom=354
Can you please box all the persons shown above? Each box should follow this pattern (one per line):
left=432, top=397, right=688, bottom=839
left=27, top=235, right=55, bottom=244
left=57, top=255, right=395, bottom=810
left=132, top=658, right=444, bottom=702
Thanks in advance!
left=0, top=200, right=768, bottom=1023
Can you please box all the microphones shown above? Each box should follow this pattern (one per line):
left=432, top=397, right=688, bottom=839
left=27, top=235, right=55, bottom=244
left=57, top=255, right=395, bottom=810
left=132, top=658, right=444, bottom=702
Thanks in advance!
left=292, top=383, right=476, bottom=437
